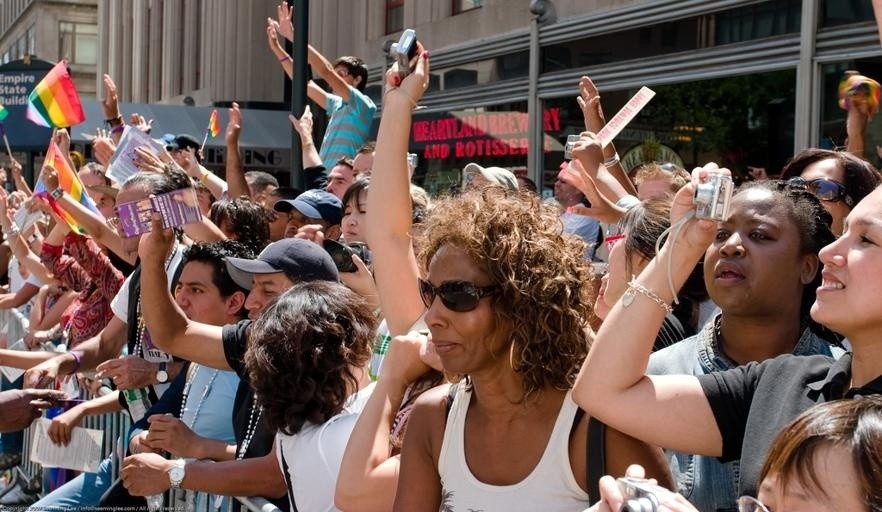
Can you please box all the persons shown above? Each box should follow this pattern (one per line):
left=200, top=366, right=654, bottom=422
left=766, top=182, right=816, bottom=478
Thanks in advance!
left=1, top=1, right=881, bottom=512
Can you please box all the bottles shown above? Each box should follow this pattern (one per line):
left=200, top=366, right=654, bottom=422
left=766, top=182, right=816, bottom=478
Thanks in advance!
left=123, top=386, right=154, bottom=425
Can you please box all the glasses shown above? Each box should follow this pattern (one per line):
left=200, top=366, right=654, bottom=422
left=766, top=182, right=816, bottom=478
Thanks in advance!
left=787, top=177, right=855, bottom=208
left=418, top=276, right=497, bottom=310
left=604, top=234, right=627, bottom=251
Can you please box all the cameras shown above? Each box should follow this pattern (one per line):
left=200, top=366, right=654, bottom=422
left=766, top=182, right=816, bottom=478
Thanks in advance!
left=389, top=29, right=416, bottom=79
left=564, top=135, right=580, bottom=160
left=323, top=238, right=372, bottom=273
left=693, top=175, right=734, bottom=222
left=616, top=477, right=675, bottom=512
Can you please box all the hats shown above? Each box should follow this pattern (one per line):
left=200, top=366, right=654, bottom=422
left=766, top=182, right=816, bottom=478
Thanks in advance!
left=275, top=189, right=344, bottom=225
left=225, top=238, right=339, bottom=291
left=463, top=162, right=519, bottom=193
left=167, top=135, right=200, bottom=151
